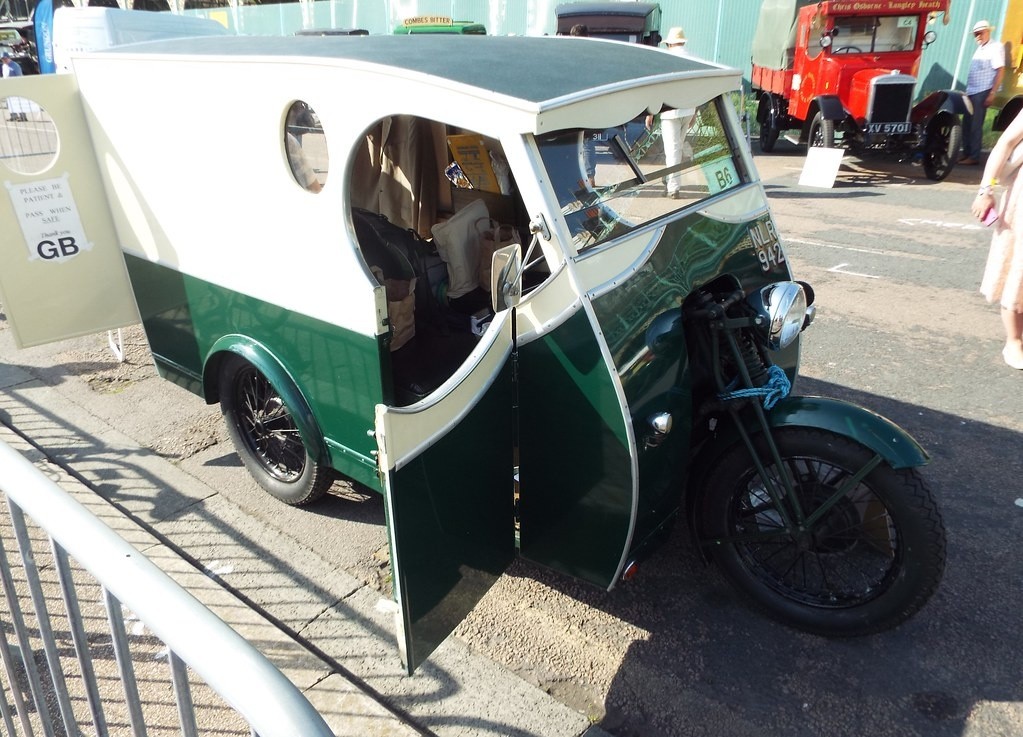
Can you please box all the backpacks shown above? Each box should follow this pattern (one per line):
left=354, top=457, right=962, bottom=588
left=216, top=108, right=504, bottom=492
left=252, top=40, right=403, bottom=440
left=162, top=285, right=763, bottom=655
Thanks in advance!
left=351, top=206, right=434, bottom=278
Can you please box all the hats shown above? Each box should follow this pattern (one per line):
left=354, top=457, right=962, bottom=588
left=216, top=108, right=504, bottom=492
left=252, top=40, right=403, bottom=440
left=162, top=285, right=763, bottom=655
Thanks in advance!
left=968, top=20, right=995, bottom=33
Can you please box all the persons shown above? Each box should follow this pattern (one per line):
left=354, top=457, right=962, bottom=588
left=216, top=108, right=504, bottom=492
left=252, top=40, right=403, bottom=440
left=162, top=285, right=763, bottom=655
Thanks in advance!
left=0, top=51, right=28, bottom=121
left=954, top=20, right=1005, bottom=165
left=645, top=27, right=697, bottom=199
left=570, top=23, right=596, bottom=188
left=970, top=106, right=1023, bottom=370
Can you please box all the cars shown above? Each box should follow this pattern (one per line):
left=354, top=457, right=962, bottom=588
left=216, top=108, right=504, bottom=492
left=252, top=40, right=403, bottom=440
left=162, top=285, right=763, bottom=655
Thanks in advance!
left=392, top=17, right=487, bottom=37
left=0, top=28, right=30, bottom=53
left=0, top=51, right=40, bottom=77
left=295, top=28, right=370, bottom=36
left=547, top=1, right=661, bottom=151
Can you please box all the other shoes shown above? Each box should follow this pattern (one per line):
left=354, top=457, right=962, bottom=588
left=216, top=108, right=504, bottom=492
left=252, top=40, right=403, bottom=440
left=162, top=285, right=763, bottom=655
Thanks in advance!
left=958, top=158, right=977, bottom=165
left=954, top=156, right=967, bottom=163
left=1002, top=338, right=1023, bottom=369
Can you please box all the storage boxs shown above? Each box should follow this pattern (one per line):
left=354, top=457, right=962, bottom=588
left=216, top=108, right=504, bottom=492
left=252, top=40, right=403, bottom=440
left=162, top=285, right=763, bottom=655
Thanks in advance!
left=451, top=185, right=519, bottom=226
left=446, top=134, right=508, bottom=193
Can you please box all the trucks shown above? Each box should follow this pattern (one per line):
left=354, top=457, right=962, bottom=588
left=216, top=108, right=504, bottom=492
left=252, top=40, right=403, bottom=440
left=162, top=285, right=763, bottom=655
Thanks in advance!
left=750, top=0, right=973, bottom=179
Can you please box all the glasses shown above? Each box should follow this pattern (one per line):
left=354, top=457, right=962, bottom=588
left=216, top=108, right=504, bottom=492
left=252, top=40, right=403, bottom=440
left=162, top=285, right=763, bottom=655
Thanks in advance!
left=974, top=33, right=982, bottom=37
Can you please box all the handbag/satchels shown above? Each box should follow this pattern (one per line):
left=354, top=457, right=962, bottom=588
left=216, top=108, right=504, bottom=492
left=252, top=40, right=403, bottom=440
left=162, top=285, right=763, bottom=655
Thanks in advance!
left=371, top=266, right=418, bottom=352
left=473, top=217, right=522, bottom=292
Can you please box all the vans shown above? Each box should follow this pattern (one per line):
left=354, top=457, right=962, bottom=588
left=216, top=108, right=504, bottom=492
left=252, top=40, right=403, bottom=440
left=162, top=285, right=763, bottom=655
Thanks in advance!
left=53, top=7, right=234, bottom=75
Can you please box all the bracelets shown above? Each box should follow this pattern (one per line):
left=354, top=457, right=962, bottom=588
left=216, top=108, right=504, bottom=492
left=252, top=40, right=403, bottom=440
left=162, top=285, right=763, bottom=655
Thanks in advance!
left=990, top=90, right=996, bottom=95
left=977, top=182, right=995, bottom=197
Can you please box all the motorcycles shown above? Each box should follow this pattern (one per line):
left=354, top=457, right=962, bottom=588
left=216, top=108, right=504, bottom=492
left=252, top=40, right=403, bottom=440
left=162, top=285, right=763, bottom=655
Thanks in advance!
left=0, top=31, right=947, bottom=678
